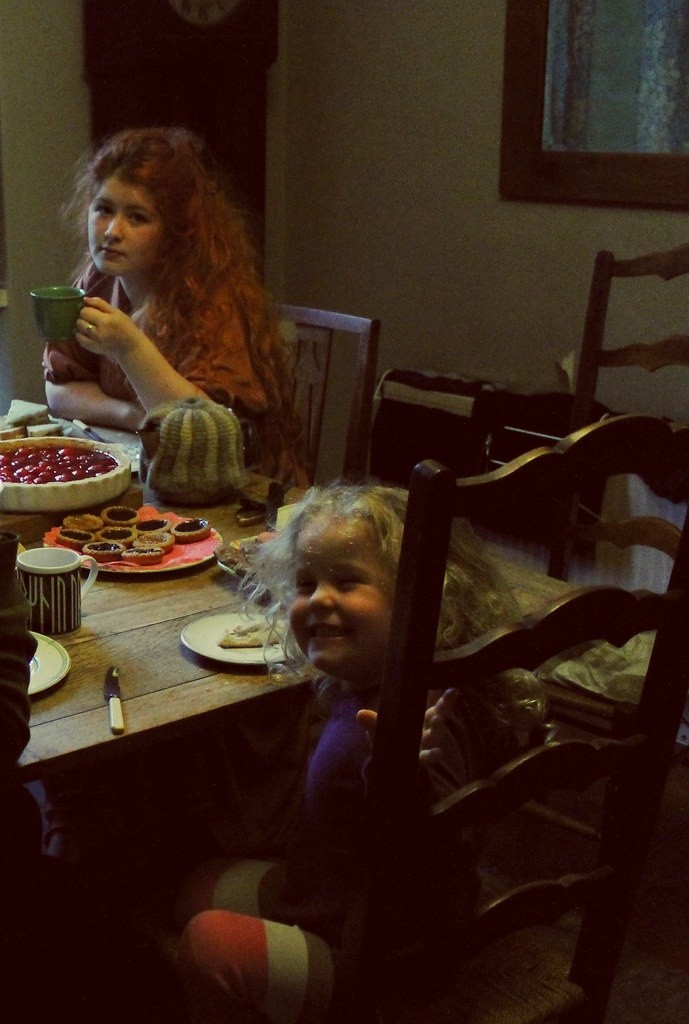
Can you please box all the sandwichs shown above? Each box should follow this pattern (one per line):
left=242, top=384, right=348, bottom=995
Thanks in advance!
left=0, top=399, right=67, bottom=440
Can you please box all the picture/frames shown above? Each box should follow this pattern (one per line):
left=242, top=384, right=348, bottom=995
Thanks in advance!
left=497, top=1, right=689, bottom=210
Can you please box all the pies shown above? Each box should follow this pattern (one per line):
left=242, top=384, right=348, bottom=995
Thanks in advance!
left=221, top=621, right=283, bottom=646
left=0, top=435, right=131, bottom=512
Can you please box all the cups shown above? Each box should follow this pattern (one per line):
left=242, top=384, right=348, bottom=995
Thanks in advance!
left=16, top=546, right=98, bottom=636
left=29, top=287, right=87, bottom=344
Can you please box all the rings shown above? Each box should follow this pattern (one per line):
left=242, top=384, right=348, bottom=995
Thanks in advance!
left=86, top=324, right=92, bottom=336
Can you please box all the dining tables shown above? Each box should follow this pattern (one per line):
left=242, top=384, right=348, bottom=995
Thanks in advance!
left=0, top=406, right=587, bottom=809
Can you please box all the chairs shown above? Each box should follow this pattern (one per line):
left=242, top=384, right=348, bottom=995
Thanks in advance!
left=321, top=416, right=689, bottom=1024
left=530, top=245, right=688, bottom=797
left=268, top=305, right=383, bottom=493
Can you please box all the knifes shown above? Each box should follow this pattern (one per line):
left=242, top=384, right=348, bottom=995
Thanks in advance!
left=103, top=665, right=125, bottom=735
left=73, top=419, right=105, bottom=443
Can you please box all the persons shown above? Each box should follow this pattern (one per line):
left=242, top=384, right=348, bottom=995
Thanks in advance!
left=0, top=532, right=42, bottom=883
left=40, top=126, right=315, bottom=491
left=132, top=480, right=542, bottom=1024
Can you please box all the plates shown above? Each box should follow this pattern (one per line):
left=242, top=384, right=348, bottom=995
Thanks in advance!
left=180, top=613, right=301, bottom=665
left=43, top=516, right=223, bottom=573
left=26, top=631, right=72, bottom=696
left=217, top=536, right=257, bottom=580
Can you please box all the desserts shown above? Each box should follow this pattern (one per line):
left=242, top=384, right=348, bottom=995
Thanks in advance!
left=55, top=506, right=211, bottom=564
